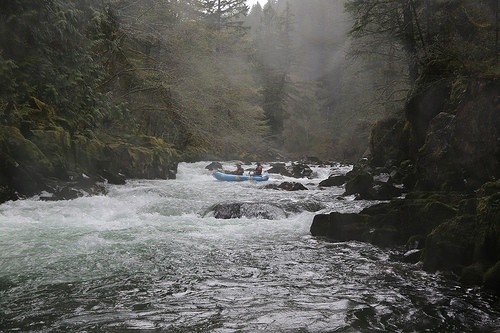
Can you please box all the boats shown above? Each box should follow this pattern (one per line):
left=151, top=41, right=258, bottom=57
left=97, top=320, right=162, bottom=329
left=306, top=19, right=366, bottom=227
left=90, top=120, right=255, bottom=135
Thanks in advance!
left=212, top=168, right=269, bottom=182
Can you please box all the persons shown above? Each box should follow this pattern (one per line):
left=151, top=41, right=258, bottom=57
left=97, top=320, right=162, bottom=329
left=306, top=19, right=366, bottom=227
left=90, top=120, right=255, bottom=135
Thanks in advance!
left=234, top=164, right=244, bottom=175
left=254, top=163, right=262, bottom=175
